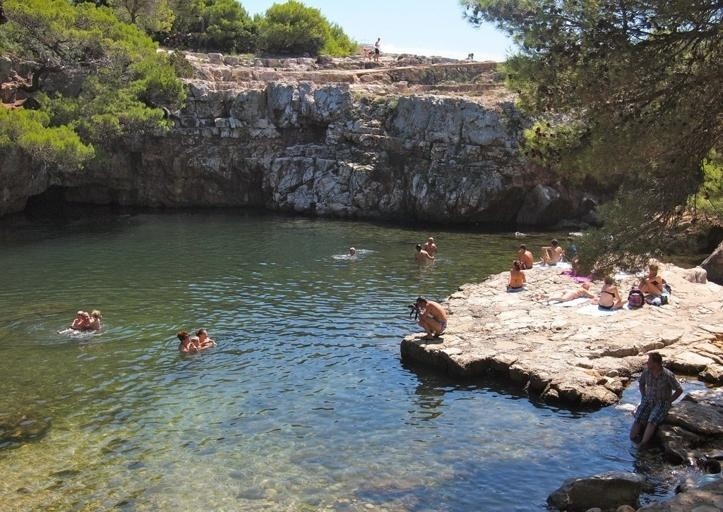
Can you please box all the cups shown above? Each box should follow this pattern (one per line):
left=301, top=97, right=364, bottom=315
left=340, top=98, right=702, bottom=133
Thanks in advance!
left=661, top=294, right=668, bottom=305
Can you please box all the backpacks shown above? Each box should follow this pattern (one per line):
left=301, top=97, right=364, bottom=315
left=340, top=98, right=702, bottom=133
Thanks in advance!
left=628, top=285, right=646, bottom=308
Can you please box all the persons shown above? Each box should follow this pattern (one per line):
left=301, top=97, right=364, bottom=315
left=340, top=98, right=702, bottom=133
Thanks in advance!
left=177, top=328, right=215, bottom=352
left=347, top=247, right=356, bottom=258
left=424, top=237, right=436, bottom=255
left=72, top=309, right=102, bottom=333
left=364, top=37, right=380, bottom=62
left=409, top=296, right=447, bottom=340
left=509, top=236, right=663, bottom=309
left=630, top=350, right=683, bottom=446
left=415, top=242, right=434, bottom=262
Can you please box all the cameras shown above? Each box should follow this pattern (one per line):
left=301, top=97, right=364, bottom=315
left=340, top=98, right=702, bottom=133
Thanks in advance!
left=408, top=303, right=418, bottom=309
